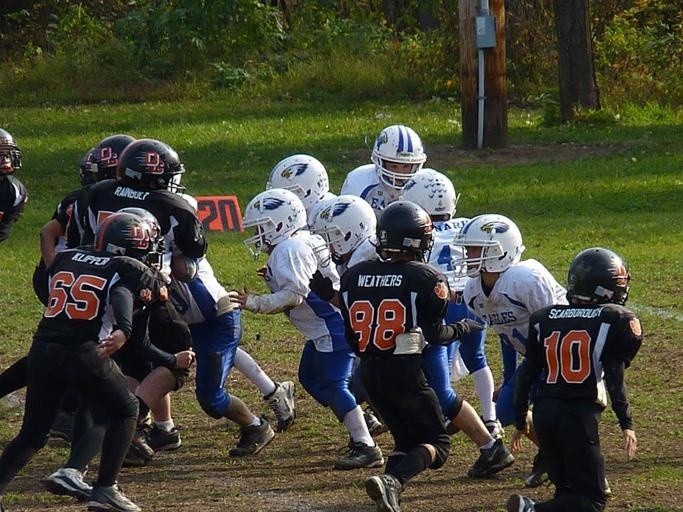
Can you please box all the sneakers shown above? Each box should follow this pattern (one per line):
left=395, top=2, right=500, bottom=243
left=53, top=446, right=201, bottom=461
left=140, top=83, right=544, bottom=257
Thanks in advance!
left=444, top=415, right=460, bottom=434
left=228, top=417, right=275, bottom=457
left=506, top=494, right=538, bottom=512
left=361, top=405, right=388, bottom=435
left=43, top=466, right=93, bottom=500
left=333, top=438, right=384, bottom=471
left=263, top=379, right=296, bottom=433
left=480, top=415, right=506, bottom=438
left=467, top=436, right=515, bottom=478
left=87, top=480, right=142, bottom=511
left=121, top=416, right=183, bottom=467
left=47, top=429, right=71, bottom=447
left=524, top=465, right=549, bottom=488
left=366, top=472, right=404, bottom=512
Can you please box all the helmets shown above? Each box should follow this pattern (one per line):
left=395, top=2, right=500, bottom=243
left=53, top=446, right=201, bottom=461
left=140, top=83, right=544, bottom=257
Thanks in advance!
left=451, top=213, right=525, bottom=278
left=311, top=193, right=379, bottom=267
left=375, top=199, right=433, bottom=266
left=373, top=122, right=426, bottom=189
left=241, top=189, right=307, bottom=259
left=80, top=133, right=186, bottom=201
left=0, top=128, right=22, bottom=177
left=94, top=206, right=165, bottom=272
left=398, top=168, right=456, bottom=221
left=565, top=247, right=631, bottom=305
left=266, top=152, right=330, bottom=206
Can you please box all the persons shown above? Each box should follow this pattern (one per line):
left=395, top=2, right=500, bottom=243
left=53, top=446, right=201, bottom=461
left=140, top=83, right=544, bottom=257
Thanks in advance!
left=229, top=126, right=643, bottom=512
left=0, top=135, right=297, bottom=511
left=2, top=129, right=28, bottom=239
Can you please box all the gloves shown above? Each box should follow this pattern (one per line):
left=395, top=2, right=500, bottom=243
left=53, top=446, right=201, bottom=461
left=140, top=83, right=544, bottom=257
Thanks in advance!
left=458, top=315, right=485, bottom=341
left=308, top=269, right=336, bottom=302
